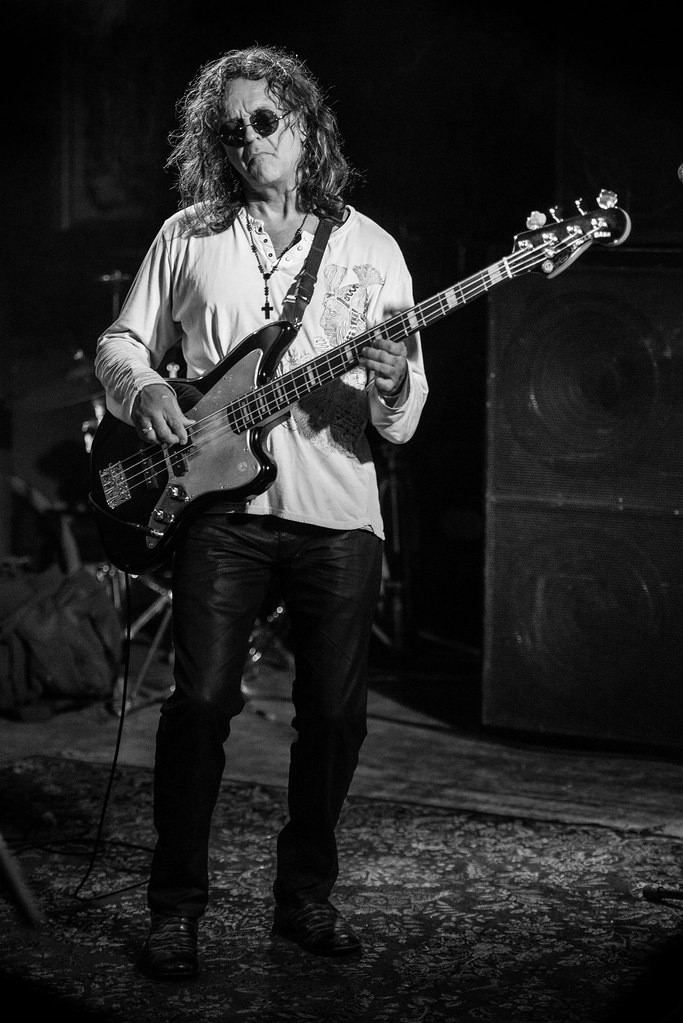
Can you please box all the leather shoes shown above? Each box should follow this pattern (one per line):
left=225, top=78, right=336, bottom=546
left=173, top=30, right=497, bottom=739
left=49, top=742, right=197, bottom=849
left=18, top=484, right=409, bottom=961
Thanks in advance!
left=140, top=911, right=199, bottom=981
left=272, top=896, right=362, bottom=955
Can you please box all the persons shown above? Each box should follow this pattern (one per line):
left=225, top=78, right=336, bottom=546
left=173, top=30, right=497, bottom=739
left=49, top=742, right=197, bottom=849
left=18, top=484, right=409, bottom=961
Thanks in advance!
left=95, top=46, right=429, bottom=979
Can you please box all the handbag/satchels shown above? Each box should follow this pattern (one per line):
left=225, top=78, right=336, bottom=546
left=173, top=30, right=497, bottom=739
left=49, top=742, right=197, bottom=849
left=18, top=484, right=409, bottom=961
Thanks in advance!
left=0, top=567, right=122, bottom=723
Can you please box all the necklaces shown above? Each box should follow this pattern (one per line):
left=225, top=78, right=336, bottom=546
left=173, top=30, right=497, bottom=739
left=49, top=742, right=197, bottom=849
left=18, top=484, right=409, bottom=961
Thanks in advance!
left=246, top=206, right=311, bottom=319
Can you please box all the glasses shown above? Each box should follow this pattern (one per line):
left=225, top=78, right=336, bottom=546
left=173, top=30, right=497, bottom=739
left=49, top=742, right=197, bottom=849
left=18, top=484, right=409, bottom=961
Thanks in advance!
left=215, top=108, right=295, bottom=146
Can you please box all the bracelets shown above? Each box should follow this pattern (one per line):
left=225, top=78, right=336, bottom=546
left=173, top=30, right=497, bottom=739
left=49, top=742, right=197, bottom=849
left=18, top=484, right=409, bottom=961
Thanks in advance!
left=382, top=365, right=408, bottom=399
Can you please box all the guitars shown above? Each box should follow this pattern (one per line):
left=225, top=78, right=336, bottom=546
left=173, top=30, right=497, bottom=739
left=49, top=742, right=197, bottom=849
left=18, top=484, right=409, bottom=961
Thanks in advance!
left=86, top=188, right=633, bottom=575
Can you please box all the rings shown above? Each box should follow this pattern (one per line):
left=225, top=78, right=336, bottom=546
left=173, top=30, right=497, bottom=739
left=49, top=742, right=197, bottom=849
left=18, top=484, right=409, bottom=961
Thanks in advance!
left=143, top=426, right=153, bottom=434
